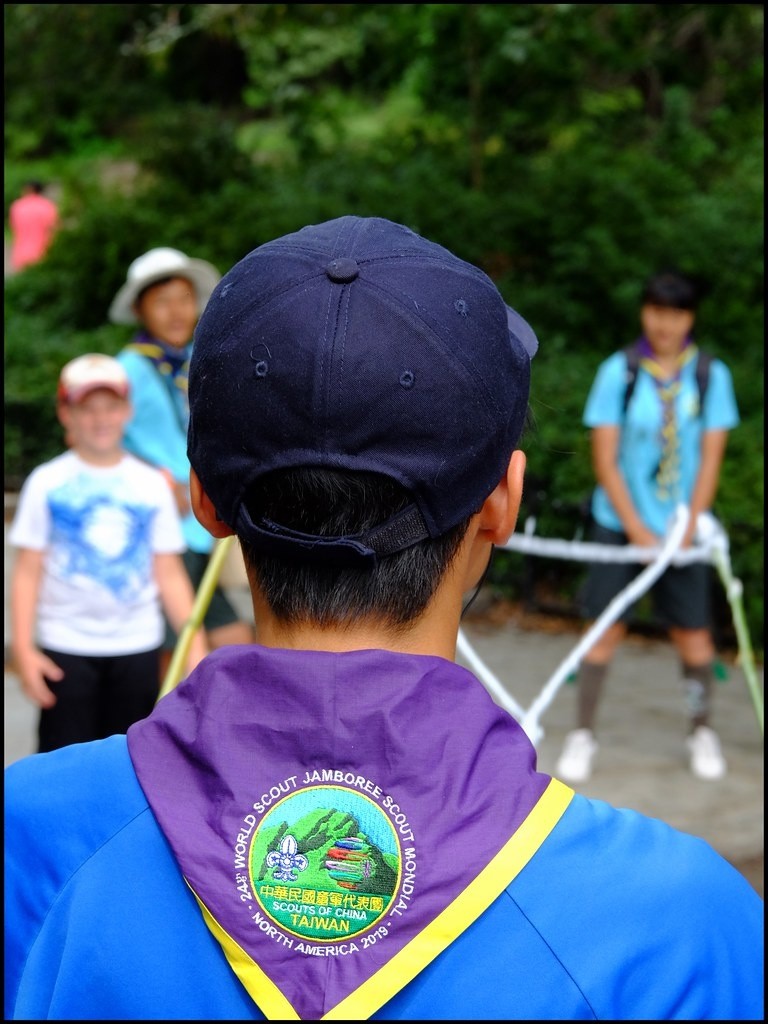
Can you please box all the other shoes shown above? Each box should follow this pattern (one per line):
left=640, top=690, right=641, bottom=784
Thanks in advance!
left=684, top=726, right=725, bottom=779
left=557, top=732, right=595, bottom=779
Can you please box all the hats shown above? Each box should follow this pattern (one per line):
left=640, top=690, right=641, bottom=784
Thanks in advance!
left=109, top=248, right=217, bottom=332
left=59, top=351, right=130, bottom=405
left=189, top=214, right=538, bottom=554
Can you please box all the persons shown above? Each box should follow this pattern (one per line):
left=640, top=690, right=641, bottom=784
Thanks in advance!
left=13, top=352, right=208, bottom=755
left=107, top=246, right=252, bottom=689
left=4, top=213, right=764, bottom=1023
left=553, top=273, right=739, bottom=783
left=9, top=181, right=59, bottom=270
